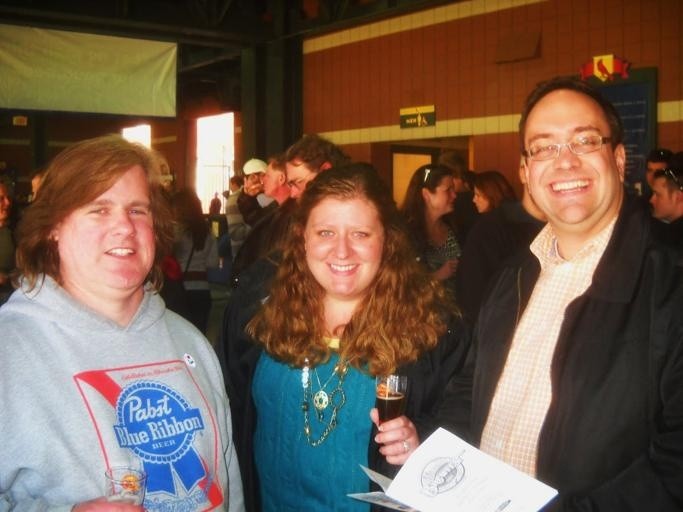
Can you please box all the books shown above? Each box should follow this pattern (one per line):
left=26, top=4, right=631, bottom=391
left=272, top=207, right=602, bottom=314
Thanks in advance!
left=345, top=425, right=560, bottom=510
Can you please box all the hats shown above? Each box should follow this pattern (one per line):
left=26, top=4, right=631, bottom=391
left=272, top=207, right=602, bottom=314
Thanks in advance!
left=243, top=158, right=268, bottom=175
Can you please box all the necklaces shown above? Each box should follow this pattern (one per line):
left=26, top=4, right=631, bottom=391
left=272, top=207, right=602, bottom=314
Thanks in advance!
left=300, top=353, right=350, bottom=447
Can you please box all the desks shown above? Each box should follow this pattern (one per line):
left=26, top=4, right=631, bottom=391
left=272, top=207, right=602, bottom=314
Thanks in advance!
left=205, top=282, right=230, bottom=346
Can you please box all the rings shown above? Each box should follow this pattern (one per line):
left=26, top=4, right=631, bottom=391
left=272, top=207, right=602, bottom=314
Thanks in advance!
left=402, top=441, right=409, bottom=451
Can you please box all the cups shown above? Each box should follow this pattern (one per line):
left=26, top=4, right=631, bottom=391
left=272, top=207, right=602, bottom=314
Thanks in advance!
left=374, top=375, right=409, bottom=422
left=105, top=468, right=146, bottom=505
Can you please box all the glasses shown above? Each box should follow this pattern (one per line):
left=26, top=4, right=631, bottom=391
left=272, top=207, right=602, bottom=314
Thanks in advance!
left=523, top=134, right=616, bottom=162
left=285, top=170, right=316, bottom=189
left=660, top=168, right=683, bottom=193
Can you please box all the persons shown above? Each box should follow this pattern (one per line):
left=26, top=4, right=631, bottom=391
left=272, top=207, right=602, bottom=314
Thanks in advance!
left=367, top=73, right=682, bottom=511
left=1, top=124, right=682, bottom=336
left=0, top=132, right=247, bottom=511
left=217, top=160, right=463, bottom=512
